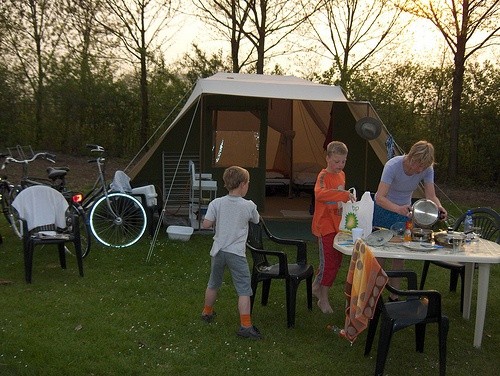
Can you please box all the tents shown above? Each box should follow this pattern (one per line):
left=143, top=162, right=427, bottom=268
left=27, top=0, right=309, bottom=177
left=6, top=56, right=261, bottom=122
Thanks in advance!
left=127, top=72, right=426, bottom=216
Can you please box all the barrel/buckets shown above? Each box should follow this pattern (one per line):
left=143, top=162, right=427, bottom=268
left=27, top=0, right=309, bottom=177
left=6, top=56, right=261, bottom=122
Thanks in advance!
left=132, top=185, right=158, bottom=208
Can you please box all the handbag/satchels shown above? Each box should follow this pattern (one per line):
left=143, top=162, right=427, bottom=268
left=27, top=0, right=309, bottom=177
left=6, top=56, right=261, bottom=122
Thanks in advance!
left=338, top=188, right=374, bottom=238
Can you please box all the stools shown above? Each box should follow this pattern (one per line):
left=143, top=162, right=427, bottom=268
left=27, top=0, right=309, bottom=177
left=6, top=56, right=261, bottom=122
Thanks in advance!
left=187, top=160, right=218, bottom=221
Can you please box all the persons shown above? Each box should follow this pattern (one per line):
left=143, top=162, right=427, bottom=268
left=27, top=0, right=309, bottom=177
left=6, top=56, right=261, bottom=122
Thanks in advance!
left=373, top=141, right=447, bottom=301
left=311, top=141, right=355, bottom=314
left=201, top=166, right=264, bottom=339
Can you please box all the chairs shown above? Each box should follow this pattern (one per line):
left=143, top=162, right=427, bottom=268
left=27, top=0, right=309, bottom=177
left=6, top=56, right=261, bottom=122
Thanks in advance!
left=246, top=215, right=314, bottom=329
left=421, top=206, right=500, bottom=294
left=107, top=170, right=163, bottom=240
left=12, top=184, right=84, bottom=284
left=346, top=238, right=449, bottom=376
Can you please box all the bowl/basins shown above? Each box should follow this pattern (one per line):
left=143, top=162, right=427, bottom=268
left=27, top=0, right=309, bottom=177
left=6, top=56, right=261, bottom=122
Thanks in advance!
left=411, top=199, right=440, bottom=227
left=166, top=226, right=194, bottom=241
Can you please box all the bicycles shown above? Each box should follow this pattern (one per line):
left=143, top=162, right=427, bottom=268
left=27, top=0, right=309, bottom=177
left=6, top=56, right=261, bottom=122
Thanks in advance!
left=0, top=142, right=149, bottom=260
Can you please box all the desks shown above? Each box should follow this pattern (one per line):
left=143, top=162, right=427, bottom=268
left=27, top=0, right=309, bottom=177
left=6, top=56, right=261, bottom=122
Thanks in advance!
left=334, top=232, right=500, bottom=348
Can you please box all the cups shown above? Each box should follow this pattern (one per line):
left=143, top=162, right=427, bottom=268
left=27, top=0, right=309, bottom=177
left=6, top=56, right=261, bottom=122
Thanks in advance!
left=448, top=236, right=465, bottom=253
left=352, top=228, right=363, bottom=242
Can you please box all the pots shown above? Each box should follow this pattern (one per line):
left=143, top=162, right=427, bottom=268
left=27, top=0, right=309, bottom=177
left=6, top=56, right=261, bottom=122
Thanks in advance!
left=411, top=228, right=432, bottom=244
left=430, top=226, right=467, bottom=247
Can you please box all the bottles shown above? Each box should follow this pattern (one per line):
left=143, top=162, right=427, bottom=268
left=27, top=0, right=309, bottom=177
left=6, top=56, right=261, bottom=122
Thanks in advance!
left=327, top=324, right=346, bottom=339
left=404, top=228, right=411, bottom=242
left=464, top=209, right=473, bottom=241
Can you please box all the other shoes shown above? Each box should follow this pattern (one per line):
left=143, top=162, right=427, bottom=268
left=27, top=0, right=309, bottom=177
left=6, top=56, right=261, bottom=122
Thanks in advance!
left=235, top=326, right=260, bottom=340
left=203, top=311, right=217, bottom=324
left=388, top=296, right=400, bottom=302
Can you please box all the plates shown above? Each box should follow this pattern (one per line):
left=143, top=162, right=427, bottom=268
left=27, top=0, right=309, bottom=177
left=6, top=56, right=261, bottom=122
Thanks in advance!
left=403, top=241, right=439, bottom=251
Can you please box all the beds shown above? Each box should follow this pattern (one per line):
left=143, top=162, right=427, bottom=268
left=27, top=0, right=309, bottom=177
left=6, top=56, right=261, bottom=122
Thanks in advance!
left=293, top=173, right=316, bottom=197
left=257, top=171, right=291, bottom=197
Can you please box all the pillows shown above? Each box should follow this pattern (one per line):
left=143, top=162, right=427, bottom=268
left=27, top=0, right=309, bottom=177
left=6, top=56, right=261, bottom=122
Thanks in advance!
left=266, top=172, right=286, bottom=179
